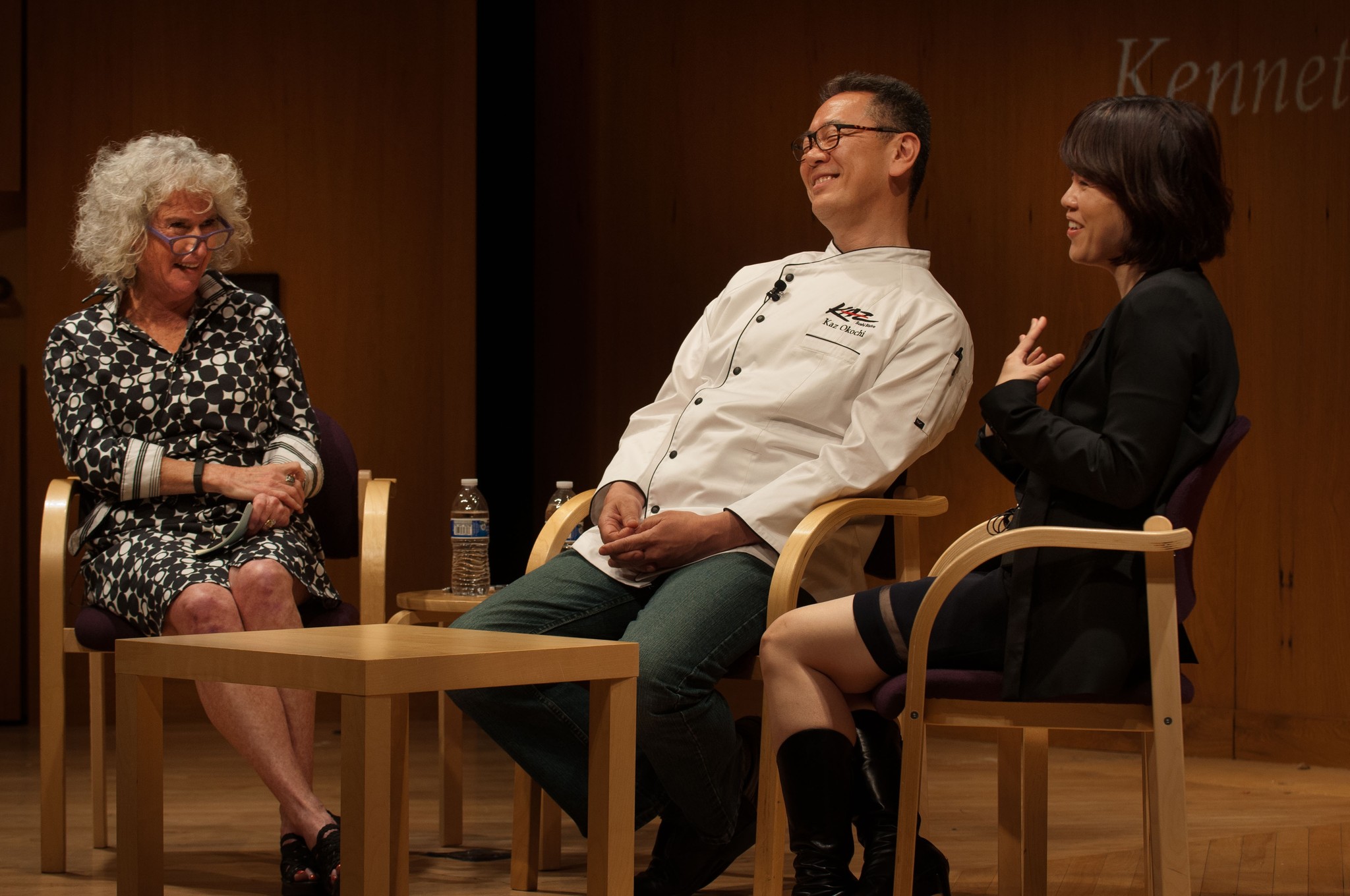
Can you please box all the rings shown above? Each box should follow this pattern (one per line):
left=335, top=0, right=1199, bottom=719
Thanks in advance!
left=285, top=474, right=295, bottom=486
left=265, top=519, right=275, bottom=528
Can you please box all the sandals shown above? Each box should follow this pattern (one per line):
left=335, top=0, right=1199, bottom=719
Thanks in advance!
left=280, top=810, right=341, bottom=896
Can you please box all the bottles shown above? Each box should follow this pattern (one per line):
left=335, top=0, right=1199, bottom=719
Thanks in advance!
left=545, top=482, right=583, bottom=554
left=451, top=479, right=491, bottom=597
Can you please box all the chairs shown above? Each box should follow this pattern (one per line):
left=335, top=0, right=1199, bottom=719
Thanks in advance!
left=507, top=459, right=948, bottom=895
left=867, top=409, right=1258, bottom=896
left=37, top=408, right=403, bottom=877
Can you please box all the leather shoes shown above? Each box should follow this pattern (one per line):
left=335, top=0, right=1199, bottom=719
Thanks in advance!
left=634, top=715, right=761, bottom=895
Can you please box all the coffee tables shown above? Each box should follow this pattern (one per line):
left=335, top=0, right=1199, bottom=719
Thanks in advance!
left=119, top=619, right=641, bottom=896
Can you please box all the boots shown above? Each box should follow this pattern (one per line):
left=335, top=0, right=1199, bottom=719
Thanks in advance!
left=776, top=708, right=950, bottom=896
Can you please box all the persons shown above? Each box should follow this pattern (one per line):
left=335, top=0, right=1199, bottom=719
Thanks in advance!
left=444, top=75, right=975, bottom=894
left=45, top=130, right=343, bottom=896
left=761, top=95, right=1241, bottom=896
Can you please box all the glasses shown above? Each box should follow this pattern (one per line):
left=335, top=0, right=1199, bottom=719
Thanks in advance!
left=144, top=214, right=234, bottom=256
left=791, top=124, right=902, bottom=162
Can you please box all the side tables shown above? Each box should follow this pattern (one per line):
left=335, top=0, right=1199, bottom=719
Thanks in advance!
left=388, top=581, right=561, bottom=869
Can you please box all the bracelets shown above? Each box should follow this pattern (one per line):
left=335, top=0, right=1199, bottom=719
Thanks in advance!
left=193, top=459, right=207, bottom=497
